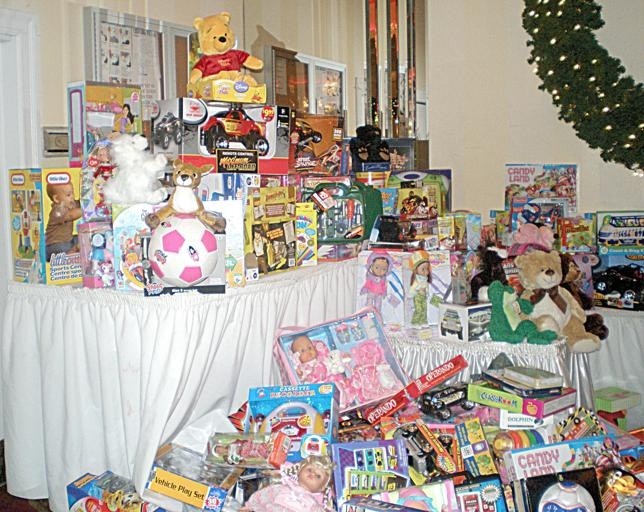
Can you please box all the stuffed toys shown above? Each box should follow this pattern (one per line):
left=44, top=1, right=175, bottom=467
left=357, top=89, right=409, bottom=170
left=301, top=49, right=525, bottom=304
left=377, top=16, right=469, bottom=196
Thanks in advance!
left=188, top=11, right=263, bottom=100
left=470, top=221, right=609, bottom=354
left=102, top=133, right=227, bottom=233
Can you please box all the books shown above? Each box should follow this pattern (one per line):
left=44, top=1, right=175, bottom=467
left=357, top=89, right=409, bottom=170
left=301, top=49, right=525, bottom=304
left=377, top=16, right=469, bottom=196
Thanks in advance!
left=466, top=364, right=581, bottom=417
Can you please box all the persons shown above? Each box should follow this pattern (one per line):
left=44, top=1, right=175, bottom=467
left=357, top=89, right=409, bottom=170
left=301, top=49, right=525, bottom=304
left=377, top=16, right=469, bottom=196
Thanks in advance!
left=44, top=180, right=86, bottom=261
left=360, top=254, right=433, bottom=326
left=242, top=454, right=336, bottom=511
left=289, top=336, right=355, bottom=413
